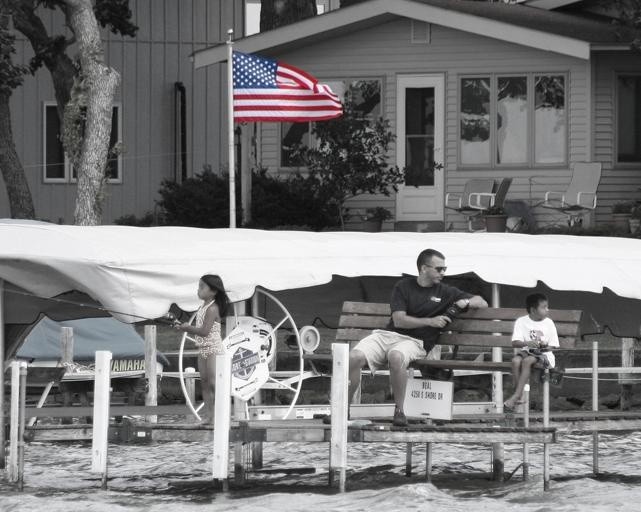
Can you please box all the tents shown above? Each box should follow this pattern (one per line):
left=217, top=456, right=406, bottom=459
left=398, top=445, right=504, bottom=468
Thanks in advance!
left=3, top=307, right=170, bottom=431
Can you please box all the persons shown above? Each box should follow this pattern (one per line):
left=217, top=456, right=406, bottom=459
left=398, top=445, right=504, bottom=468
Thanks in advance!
left=503, top=293, right=560, bottom=413
left=322, top=249, right=488, bottom=426
left=177, top=274, right=231, bottom=428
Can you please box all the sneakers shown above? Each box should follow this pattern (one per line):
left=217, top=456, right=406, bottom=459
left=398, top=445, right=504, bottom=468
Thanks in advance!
left=393, top=411, right=407, bottom=426
left=323, top=412, right=351, bottom=424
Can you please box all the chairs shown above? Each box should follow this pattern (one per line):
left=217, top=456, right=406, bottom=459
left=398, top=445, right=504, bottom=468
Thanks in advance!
left=541, top=163, right=602, bottom=227
left=445, top=178, right=496, bottom=231
left=303, top=301, right=584, bottom=492
left=468, top=177, right=513, bottom=231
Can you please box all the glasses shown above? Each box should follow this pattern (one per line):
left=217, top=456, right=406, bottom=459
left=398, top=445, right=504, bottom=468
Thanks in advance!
left=424, top=263, right=447, bottom=272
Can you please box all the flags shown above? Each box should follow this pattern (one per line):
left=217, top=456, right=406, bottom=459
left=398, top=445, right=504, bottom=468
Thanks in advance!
left=230, top=49, right=344, bottom=121
